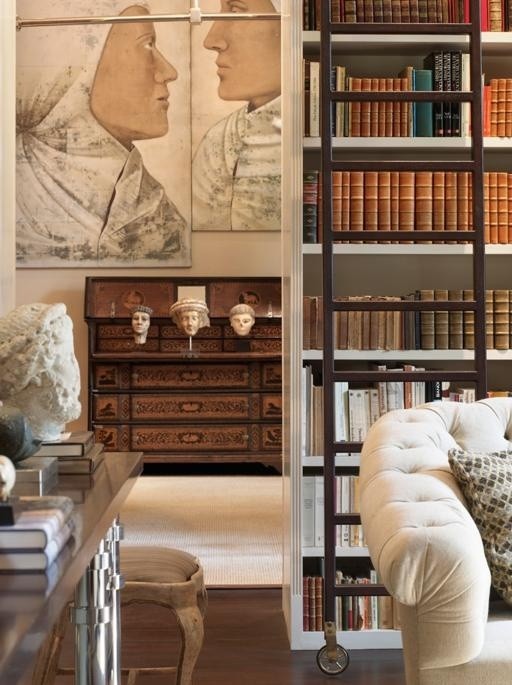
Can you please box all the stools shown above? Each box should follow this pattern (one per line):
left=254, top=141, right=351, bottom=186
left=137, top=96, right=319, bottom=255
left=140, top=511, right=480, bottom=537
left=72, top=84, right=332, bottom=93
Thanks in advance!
left=31, top=544, right=208, bottom=685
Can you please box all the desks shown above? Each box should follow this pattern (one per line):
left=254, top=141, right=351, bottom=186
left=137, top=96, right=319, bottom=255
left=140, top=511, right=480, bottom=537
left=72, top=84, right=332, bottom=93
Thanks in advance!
left=0, top=452, right=144, bottom=684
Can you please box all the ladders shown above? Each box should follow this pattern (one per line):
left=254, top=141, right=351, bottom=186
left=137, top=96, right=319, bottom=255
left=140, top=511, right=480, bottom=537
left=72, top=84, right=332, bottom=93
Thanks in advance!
left=316, top=0, right=489, bottom=676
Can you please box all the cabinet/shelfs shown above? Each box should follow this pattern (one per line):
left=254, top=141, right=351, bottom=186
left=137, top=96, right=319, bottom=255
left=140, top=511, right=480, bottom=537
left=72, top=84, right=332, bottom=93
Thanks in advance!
left=283, top=0, right=512, bottom=650
left=81, top=276, right=283, bottom=480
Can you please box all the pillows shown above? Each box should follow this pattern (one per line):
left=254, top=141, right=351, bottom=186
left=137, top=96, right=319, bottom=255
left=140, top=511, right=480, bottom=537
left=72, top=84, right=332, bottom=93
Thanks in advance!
left=447, top=446, right=512, bottom=610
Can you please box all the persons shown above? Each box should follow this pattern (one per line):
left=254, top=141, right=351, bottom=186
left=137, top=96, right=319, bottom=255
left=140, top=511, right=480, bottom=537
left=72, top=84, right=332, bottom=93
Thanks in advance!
left=1, top=300, right=83, bottom=442
left=128, top=306, right=152, bottom=345
left=229, top=304, right=256, bottom=336
left=169, top=298, right=210, bottom=336
left=189, top=0, right=284, bottom=231
left=18, top=0, right=192, bottom=264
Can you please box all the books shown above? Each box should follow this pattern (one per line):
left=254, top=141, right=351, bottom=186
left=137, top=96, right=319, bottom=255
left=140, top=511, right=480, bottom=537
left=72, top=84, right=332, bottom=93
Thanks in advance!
left=305, top=290, right=512, bottom=350
left=304, top=172, right=512, bottom=246
left=0, top=495, right=74, bottom=551
left=0, top=589, right=52, bottom=614
left=0, top=516, right=75, bottom=574
left=14, top=456, right=60, bottom=484
left=305, top=362, right=511, bottom=633
left=34, top=430, right=96, bottom=457
left=50, top=486, right=94, bottom=504
left=0, top=524, right=84, bottom=595
left=59, top=460, right=105, bottom=489
left=12, top=475, right=60, bottom=496
left=307, top=0, right=512, bottom=33
left=56, top=442, right=106, bottom=476
left=305, top=48, right=511, bottom=137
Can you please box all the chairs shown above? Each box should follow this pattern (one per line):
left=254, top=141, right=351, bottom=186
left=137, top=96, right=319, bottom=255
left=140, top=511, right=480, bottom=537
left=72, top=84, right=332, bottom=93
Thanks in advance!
left=358, top=395, right=512, bottom=684
